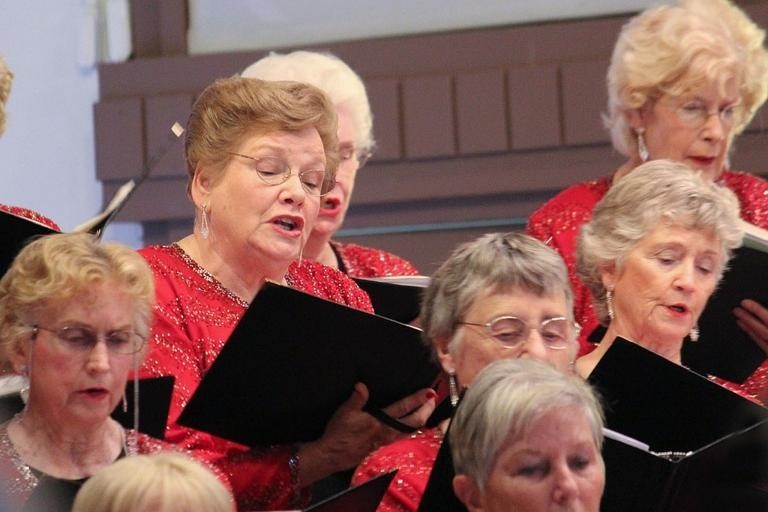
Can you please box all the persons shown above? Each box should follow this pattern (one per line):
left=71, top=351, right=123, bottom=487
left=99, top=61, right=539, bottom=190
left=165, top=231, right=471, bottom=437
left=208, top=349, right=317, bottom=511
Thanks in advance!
left=243, top=51, right=422, bottom=278
left=451, top=358, right=609, bottom=512
left=524, top=1, right=768, bottom=355
left=0, top=233, right=237, bottom=512
left=349, top=231, right=582, bottom=512
left=2, top=59, right=63, bottom=233
left=69, top=455, right=232, bottom=510
left=128, top=77, right=440, bottom=512
left=569, top=159, right=767, bottom=409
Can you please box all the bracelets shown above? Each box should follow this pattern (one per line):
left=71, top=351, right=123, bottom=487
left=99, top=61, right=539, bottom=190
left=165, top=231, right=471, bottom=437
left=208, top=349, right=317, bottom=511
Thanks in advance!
left=288, top=449, right=307, bottom=487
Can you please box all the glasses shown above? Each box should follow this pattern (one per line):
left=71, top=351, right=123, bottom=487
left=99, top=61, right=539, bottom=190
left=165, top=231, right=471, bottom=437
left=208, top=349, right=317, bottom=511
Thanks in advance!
left=455, top=316, right=583, bottom=349
left=33, top=325, right=149, bottom=355
left=231, top=152, right=334, bottom=196
left=651, top=98, right=746, bottom=127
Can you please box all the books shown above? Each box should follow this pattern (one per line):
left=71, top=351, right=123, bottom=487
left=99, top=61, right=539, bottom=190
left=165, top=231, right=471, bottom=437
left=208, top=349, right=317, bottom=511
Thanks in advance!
left=0, top=175, right=140, bottom=276
left=586, top=338, right=768, bottom=512
left=178, top=282, right=466, bottom=450
left=3, top=373, right=174, bottom=443
left=346, top=275, right=432, bottom=323
left=302, top=469, right=399, bottom=510
left=587, top=217, right=768, bottom=383
